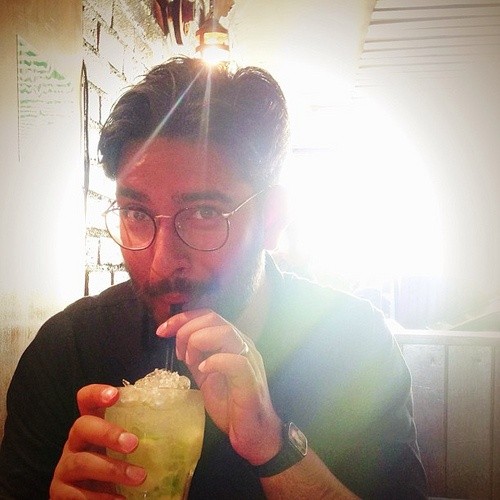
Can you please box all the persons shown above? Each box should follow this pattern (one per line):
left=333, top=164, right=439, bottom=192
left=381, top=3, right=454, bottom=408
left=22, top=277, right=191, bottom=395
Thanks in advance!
left=0, top=59, right=428, bottom=500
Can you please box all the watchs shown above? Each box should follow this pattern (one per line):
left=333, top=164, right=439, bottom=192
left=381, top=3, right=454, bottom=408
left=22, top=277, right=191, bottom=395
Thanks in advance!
left=252, top=416, right=308, bottom=478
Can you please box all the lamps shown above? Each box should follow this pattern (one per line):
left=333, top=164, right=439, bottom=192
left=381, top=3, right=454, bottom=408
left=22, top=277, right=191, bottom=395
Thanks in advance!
left=154, top=0, right=235, bottom=72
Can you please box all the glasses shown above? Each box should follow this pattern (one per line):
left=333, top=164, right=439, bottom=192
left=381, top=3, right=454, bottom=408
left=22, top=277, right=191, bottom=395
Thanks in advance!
left=101, top=179, right=279, bottom=251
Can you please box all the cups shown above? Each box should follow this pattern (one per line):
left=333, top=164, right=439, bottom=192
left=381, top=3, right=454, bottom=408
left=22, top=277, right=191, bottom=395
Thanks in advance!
left=102, top=386, right=205, bottom=500
